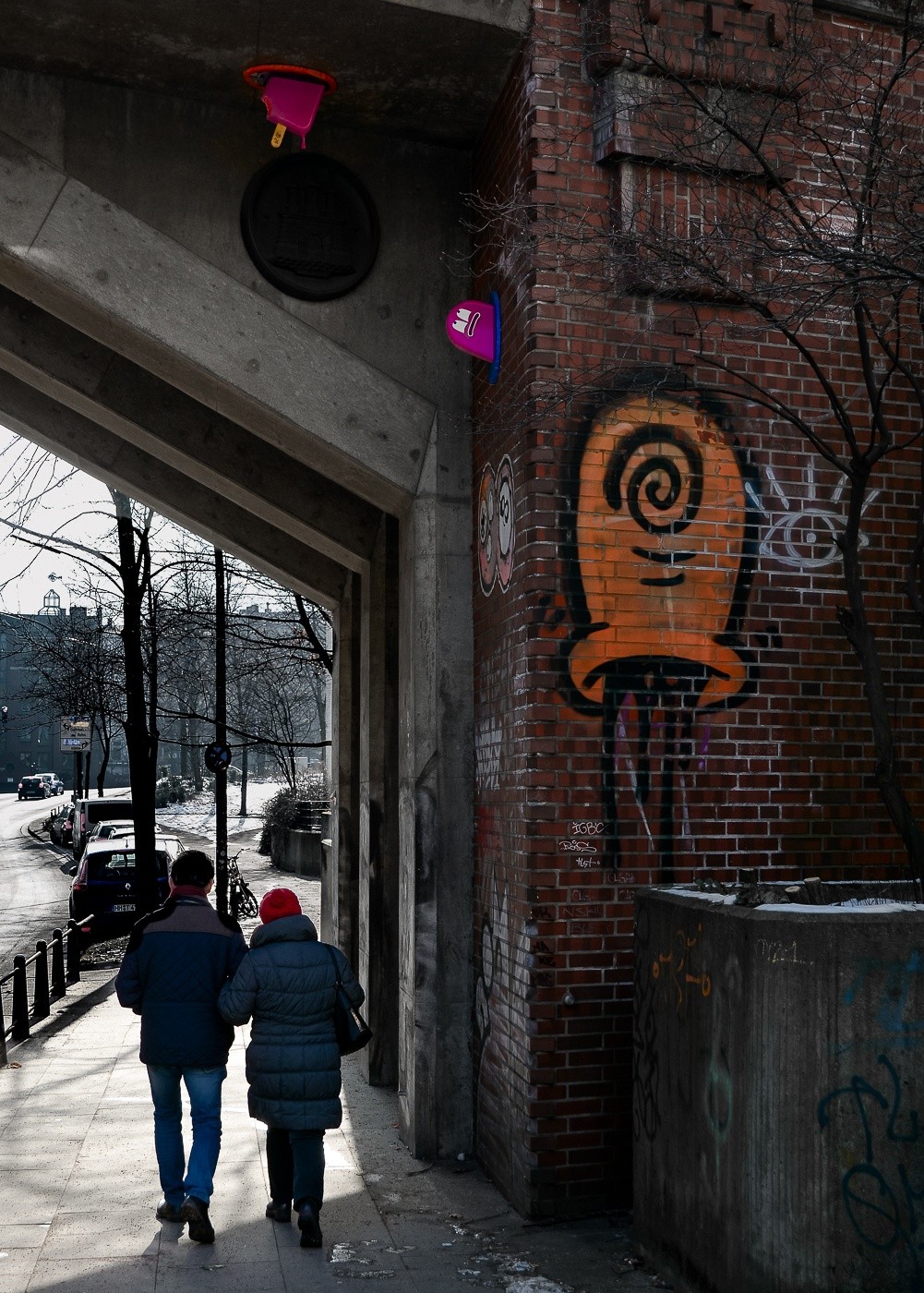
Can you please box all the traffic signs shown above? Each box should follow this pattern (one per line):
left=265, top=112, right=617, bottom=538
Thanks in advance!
left=60, top=714, right=90, bottom=751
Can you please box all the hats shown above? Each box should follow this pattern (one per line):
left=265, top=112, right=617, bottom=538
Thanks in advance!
left=260, top=888, right=302, bottom=926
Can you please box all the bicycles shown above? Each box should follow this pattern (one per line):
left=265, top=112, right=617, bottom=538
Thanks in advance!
left=225, top=848, right=259, bottom=920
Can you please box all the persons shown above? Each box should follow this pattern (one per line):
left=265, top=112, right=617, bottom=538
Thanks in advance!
left=115, top=850, right=252, bottom=1244
left=217, top=888, right=366, bottom=1250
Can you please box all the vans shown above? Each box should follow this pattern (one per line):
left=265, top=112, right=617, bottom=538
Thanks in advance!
left=72, top=799, right=133, bottom=858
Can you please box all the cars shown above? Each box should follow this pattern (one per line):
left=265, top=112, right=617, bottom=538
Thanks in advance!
left=108, top=824, right=162, bottom=838
left=69, top=837, right=174, bottom=950
left=18, top=775, right=51, bottom=798
left=87, top=820, right=136, bottom=842
left=51, top=805, right=75, bottom=847
left=106, top=833, right=185, bottom=888
left=34, top=772, right=65, bottom=796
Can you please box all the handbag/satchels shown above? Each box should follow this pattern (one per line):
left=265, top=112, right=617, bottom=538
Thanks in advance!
left=330, top=985, right=373, bottom=1056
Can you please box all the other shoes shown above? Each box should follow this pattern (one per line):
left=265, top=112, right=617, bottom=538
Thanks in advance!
left=157, top=1200, right=187, bottom=1223
left=182, top=1195, right=215, bottom=1245
left=266, top=1199, right=291, bottom=1223
left=300, top=1226, right=323, bottom=1249
